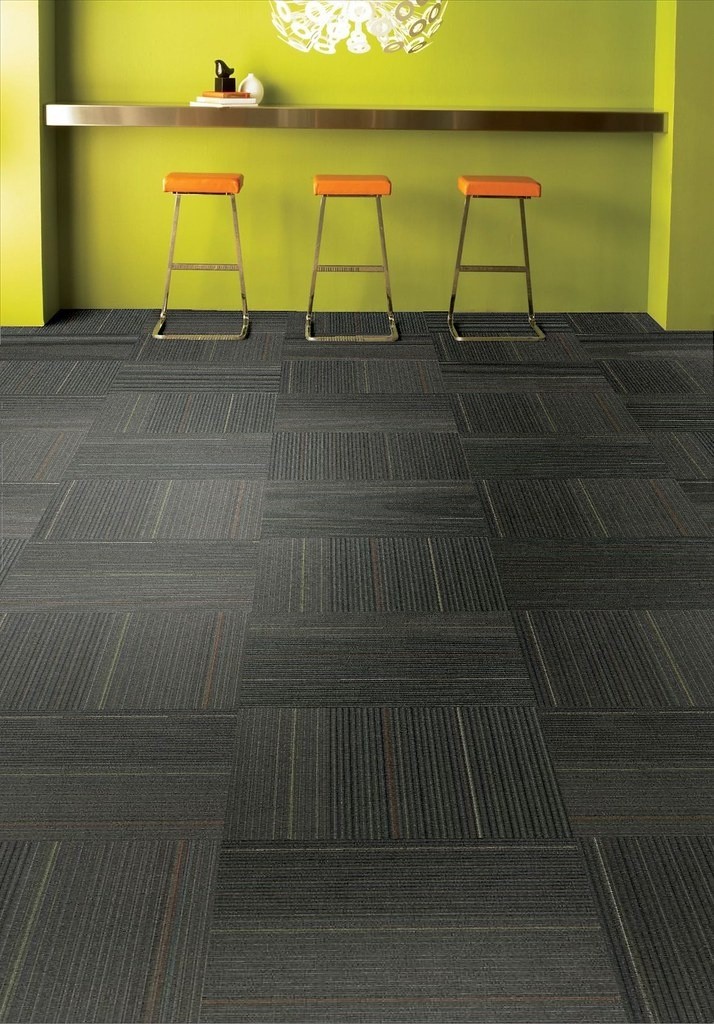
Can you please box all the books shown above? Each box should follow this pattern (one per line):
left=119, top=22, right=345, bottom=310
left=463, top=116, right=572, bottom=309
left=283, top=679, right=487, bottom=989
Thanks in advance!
left=189, top=96, right=258, bottom=109
left=203, top=91, right=250, bottom=98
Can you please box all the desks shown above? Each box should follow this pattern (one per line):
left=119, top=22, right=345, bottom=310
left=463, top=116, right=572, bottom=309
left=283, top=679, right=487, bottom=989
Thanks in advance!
left=44, top=102, right=669, bottom=134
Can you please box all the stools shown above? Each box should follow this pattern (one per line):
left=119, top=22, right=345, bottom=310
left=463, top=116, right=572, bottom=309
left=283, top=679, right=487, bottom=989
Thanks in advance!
left=304, top=173, right=400, bottom=344
left=151, top=173, right=250, bottom=341
left=447, top=174, right=545, bottom=343
